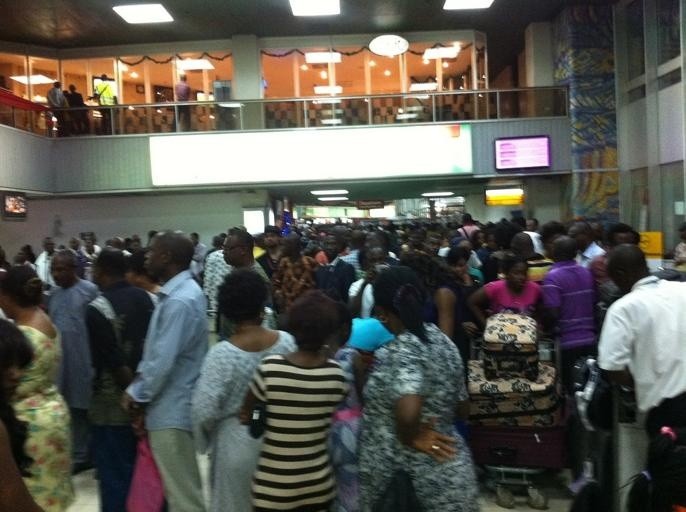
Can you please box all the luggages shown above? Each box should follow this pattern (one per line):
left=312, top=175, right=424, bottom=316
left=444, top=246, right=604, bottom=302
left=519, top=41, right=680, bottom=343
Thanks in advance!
left=467, top=314, right=576, bottom=468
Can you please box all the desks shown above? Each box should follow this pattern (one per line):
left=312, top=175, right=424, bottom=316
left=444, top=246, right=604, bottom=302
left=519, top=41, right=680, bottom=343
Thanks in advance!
left=578, top=356, right=651, bottom=512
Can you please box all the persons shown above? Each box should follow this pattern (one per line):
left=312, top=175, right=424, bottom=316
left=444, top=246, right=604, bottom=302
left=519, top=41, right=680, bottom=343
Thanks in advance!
left=1, top=214, right=686, bottom=512
left=43, top=70, right=123, bottom=137
left=174, top=74, right=196, bottom=131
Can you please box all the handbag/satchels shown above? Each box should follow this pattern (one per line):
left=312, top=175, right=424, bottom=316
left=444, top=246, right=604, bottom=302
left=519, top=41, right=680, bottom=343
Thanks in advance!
left=127, top=437, right=163, bottom=512
left=89, top=367, right=142, bottom=426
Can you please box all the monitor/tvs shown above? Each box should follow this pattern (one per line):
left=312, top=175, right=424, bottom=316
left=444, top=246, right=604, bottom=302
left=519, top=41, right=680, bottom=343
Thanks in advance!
left=1, top=190, right=27, bottom=222
left=492, top=134, right=552, bottom=172
left=196, top=91, right=214, bottom=103
left=92, top=76, right=117, bottom=99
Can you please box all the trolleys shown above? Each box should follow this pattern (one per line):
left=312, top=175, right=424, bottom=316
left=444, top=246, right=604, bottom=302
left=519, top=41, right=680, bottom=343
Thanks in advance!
left=469, top=332, right=572, bottom=510
